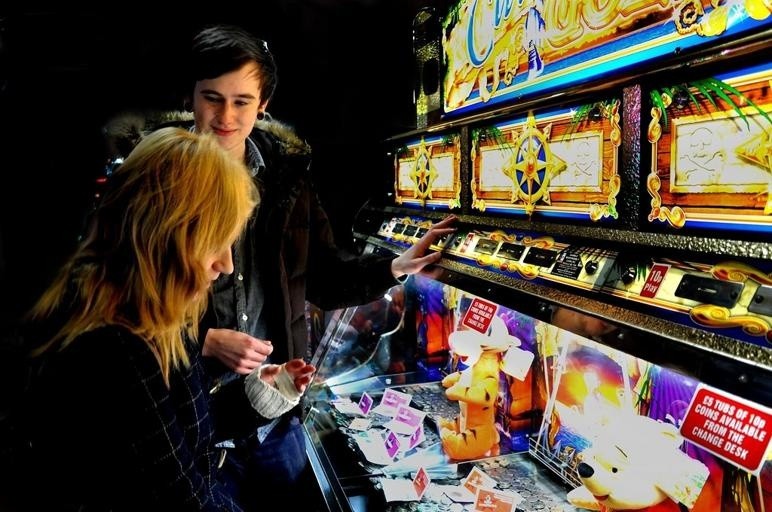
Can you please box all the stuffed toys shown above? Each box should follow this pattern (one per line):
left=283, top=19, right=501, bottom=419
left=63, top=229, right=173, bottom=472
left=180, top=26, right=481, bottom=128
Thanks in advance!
left=431, top=314, right=522, bottom=462
left=567, top=415, right=710, bottom=511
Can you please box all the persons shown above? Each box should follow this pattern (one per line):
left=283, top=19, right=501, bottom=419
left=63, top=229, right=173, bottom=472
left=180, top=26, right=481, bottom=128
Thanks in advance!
left=3, top=127, right=258, bottom=512
left=146, top=25, right=460, bottom=507
